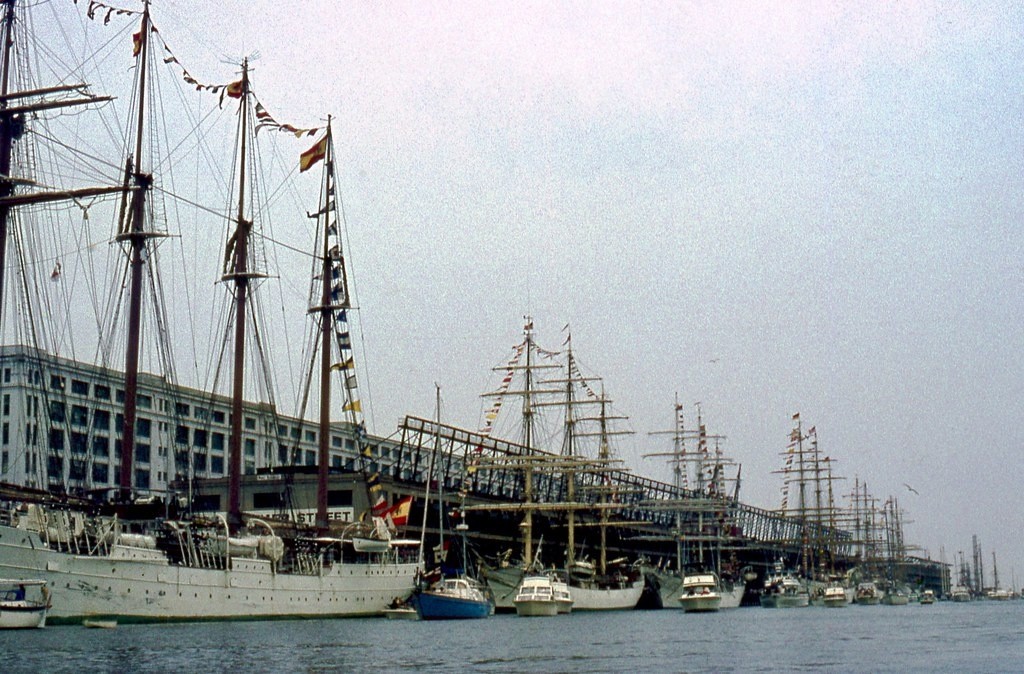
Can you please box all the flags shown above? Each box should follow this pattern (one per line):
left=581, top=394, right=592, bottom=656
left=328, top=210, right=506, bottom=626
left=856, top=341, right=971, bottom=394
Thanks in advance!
left=338, top=331, right=352, bottom=349
left=369, top=472, right=382, bottom=492
left=777, top=411, right=836, bottom=527
left=300, top=133, right=328, bottom=173
left=390, top=496, right=413, bottom=527
left=670, top=404, right=729, bottom=499
left=330, top=280, right=345, bottom=301
left=342, top=399, right=361, bottom=412
left=327, top=220, right=337, bottom=235
left=448, top=321, right=534, bottom=519
left=370, top=495, right=388, bottom=511
left=344, top=375, right=357, bottom=389
left=327, top=356, right=354, bottom=370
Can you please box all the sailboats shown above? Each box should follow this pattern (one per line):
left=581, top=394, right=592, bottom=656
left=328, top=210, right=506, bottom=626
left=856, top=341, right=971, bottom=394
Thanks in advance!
left=0, top=1, right=424, bottom=616
left=443, top=318, right=1014, bottom=611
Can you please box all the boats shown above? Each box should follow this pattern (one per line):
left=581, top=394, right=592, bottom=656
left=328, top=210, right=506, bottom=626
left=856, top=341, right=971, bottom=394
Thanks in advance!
left=1, top=579, right=52, bottom=629
left=677, top=575, right=721, bottom=614
left=411, top=579, right=491, bottom=618
left=514, top=577, right=573, bottom=616
